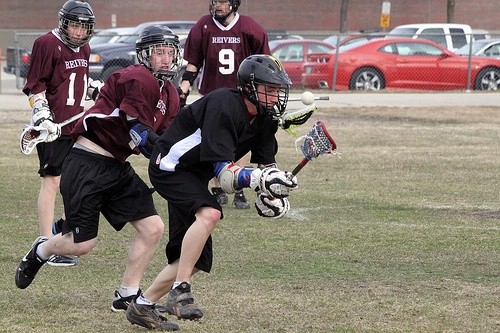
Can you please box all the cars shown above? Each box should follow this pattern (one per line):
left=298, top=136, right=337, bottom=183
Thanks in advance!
left=268, top=40, right=337, bottom=89
left=2, top=20, right=492, bottom=87
left=454, top=38, right=500, bottom=59
left=299, top=37, right=500, bottom=93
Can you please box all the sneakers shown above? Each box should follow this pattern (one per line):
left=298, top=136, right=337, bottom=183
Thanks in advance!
left=111, top=288, right=166, bottom=313
left=47, top=254, right=79, bottom=267
left=166, top=281, right=203, bottom=321
left=126, top=289, right=180, bottom=332
left=15, top=236, right=49, bottom=289
left=52, top=218, right=65, bottom=235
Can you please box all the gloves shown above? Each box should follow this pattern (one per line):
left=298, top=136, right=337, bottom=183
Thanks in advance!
left=39, top=120, right=61, bottom=143
left=250, top=168, right=298, bottom=199
left=254, top=193, right=290, bottom=219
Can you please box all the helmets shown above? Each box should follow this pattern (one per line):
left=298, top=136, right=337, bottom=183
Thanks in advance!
left=237, top=54, right=293, bottom=89
left=136, top=24, right=179, bottom=48
left=59, top=0, right=96, bottom=23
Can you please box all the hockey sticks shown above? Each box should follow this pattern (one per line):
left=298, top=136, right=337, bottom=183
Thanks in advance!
left=279, top=108, right=317, bottom=135
left=20, top=112, right=84, bottom=156
left=287, top=121, right=337, bottom=182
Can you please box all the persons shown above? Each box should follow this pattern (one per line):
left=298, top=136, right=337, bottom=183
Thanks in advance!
left=15, top=24, right=181, bottom=321
left=22, top=0, right=106, bottom=267
left=126, top=55, right=299, bottom=332
left=178, top=0, right=273, bottom=209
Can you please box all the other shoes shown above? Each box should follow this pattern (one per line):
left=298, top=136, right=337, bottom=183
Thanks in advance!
left=211, top=187, right=228, bottom=204
left=233, top=191, right=250, bottom=209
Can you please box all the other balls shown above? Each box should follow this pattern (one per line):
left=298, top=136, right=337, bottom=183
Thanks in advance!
left=301, top=91, right=314, bottom=105
